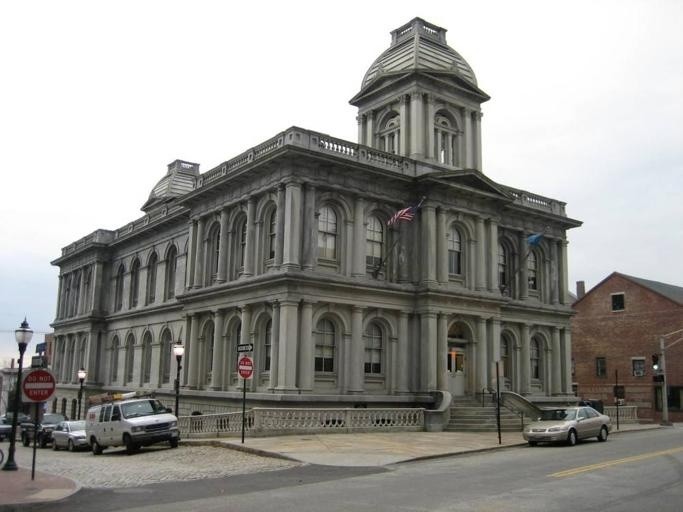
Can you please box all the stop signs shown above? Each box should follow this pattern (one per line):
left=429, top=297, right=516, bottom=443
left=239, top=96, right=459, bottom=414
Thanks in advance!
left=237, top=356, right=254, bottom=380
left=24, top=368, right=56, bottom=401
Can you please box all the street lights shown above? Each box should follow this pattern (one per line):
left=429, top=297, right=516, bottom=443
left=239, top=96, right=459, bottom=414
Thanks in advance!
left=172, top=341, right=184, bottom=418
left=77, top=368, right=86, bottom=418
left=3, top=317, right=33, bottom=470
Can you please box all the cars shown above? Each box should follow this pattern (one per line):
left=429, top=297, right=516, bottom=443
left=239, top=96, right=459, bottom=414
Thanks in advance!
left=523, top=407, right=612, bottom=446
left=0, top=412, right=87, bottom=451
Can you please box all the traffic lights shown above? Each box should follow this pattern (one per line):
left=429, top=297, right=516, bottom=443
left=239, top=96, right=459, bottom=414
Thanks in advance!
left=652, top=354, right=659, bottom=370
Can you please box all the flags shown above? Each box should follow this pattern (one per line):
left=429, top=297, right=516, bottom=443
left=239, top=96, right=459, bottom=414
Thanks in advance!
left=385, top=204, right=416, bottom=224
left=525, top=231, right=542, bottom=247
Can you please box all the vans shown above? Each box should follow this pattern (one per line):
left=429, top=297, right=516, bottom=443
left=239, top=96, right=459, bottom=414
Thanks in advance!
left=83, top=399, right=181, bottom=455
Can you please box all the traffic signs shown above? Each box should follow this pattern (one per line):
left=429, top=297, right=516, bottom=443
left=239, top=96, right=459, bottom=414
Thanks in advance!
left=237, top=343, right=254, bottom=352
